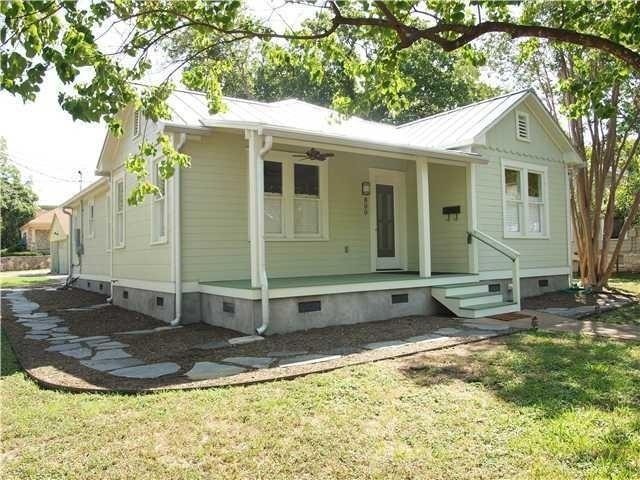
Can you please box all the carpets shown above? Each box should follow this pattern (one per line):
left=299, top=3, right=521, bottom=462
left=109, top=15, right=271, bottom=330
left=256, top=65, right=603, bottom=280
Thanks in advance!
left=487, top=313, right=531, bottom=322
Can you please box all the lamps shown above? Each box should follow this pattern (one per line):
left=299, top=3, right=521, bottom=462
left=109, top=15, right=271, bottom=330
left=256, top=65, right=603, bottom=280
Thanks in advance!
left=362, top=181, right=370, bottom=196
left=364, top=197, right=368, bottom=215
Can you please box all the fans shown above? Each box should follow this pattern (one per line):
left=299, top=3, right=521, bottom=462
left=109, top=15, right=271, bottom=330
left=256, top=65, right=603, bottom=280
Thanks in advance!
left=292, top=148, right=337, bottom=163
